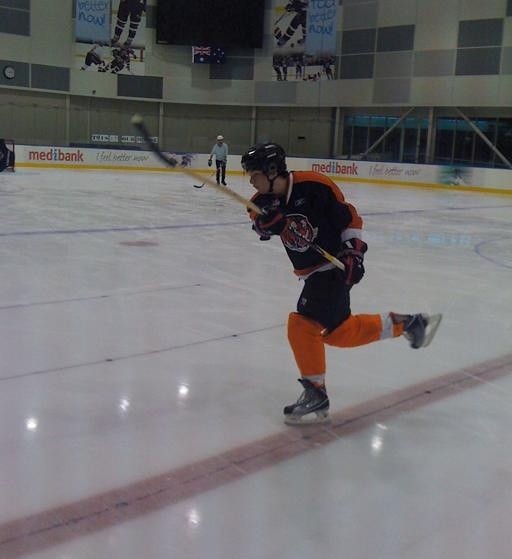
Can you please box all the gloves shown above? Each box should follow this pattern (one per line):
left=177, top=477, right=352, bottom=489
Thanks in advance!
left=336, top=238, right=367, bottom=286
left=252, top=205, right=287, bottom=237
left=208, top=159, right=212, bottom=166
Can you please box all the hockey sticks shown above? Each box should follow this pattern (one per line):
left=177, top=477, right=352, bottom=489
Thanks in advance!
left=193, top=163, right=224, bottom=188
left=131, top=114, right=345, bottom=270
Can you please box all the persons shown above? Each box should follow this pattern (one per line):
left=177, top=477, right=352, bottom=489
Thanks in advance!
left=79, top=45, right=136, bottom=74
left=239, top=143, right=429, bottom=414
left=273, top=55, right=338, bottom=81
left=208, top=134, right=228, bottom=185
left=275, top=0, right=308, bottom=47
left=111, top=0, right=152, bottom=46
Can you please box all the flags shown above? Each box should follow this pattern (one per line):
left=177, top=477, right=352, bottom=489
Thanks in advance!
left=192, top=46, right=226, bottom=64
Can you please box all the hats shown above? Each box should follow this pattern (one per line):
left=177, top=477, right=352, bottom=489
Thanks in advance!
left=217, top=135, right=224, bottom=140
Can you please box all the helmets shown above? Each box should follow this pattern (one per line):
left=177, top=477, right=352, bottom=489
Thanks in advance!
left=241, top=143, right=286, bottom=170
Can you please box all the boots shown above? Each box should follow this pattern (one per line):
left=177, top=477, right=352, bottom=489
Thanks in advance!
left=292, top=379, right=329, bottom=415
left=391, top=312, right=427, bottom=347
